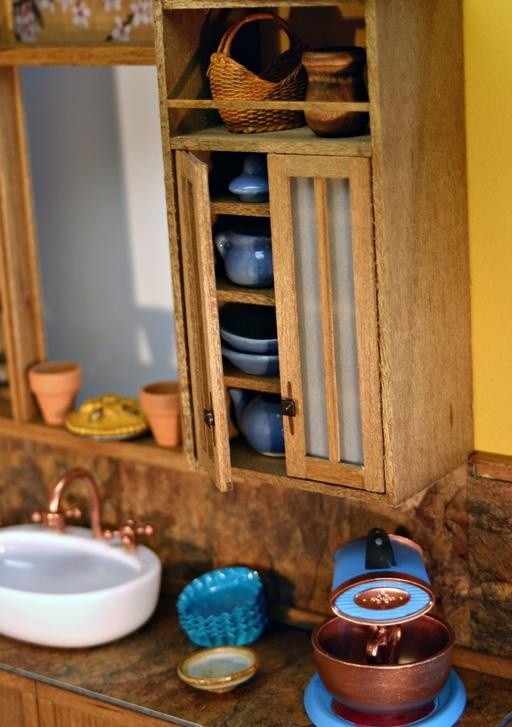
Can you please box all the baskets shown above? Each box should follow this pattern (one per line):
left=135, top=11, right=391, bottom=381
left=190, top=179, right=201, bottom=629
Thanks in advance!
left=206, top=13, right=312, bottom=134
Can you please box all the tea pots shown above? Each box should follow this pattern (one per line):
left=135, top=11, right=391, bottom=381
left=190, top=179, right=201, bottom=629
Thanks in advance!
left=227, top=387, right=287, bottom=459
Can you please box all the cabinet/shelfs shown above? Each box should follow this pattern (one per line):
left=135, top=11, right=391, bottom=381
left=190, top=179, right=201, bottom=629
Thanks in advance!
left=0, top=669, right=187, bottom=727
left=153, top=1, right=466, bottom=512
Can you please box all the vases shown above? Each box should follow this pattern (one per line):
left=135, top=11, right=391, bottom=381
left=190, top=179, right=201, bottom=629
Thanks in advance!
left=29, top=361, right=85, bottom=423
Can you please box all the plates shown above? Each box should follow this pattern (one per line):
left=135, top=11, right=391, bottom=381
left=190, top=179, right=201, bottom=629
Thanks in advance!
left=176, top=565, right=267, bottom=693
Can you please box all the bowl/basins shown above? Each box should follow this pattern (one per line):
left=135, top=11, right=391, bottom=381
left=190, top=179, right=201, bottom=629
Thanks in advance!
left=217, top=305, right=280, bottom=379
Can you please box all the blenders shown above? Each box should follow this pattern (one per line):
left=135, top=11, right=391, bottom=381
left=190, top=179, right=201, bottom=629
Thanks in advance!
left=303, top=526, right=468, bottom=727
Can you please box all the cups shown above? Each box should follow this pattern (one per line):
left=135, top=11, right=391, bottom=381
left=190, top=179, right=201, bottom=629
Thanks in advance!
left=27, top=359, right=82, bottom=429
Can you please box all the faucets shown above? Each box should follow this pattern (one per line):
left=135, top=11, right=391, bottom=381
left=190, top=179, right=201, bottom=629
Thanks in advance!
left=46, top=466, right=114, bottom=539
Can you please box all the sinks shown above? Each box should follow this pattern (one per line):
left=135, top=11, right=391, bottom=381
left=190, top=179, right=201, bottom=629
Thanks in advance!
left=0, top=523, right=162, bottom=649
